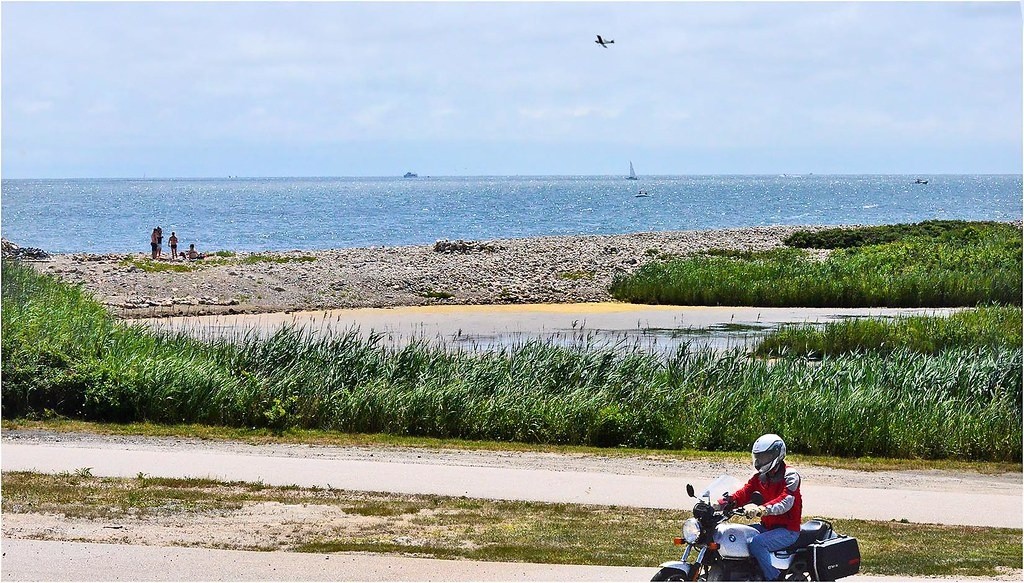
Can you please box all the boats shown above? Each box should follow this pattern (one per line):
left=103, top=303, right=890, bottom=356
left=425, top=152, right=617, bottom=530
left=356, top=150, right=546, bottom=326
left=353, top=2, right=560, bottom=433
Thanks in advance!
left=915, top=179, right=929, bottom=184
left=635, top=194, right=652, bottom=197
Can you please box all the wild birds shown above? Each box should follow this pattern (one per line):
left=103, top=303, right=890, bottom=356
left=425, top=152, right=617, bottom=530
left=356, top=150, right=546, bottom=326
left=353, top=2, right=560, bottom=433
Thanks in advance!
left=595, top=35, right=614, bottom=48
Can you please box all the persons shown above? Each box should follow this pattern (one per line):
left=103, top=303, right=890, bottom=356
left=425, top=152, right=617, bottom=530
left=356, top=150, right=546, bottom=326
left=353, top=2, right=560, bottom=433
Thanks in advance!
left=168, top=232, right=178, bottom=259
left=151, top=226, right=164, bottom=259
left=187, top=244, right=199, bottom=260
left=709, top=434, right=803, bottom=582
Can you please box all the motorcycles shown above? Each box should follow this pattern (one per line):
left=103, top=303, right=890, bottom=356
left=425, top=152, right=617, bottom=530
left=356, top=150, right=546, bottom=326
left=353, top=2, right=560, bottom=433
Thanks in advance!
left=650, top=474, right=860, bottom=582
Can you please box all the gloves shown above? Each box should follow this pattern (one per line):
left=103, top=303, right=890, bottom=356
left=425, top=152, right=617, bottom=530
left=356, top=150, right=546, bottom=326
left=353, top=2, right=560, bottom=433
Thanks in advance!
left=744, top=503, right=759, bottom=519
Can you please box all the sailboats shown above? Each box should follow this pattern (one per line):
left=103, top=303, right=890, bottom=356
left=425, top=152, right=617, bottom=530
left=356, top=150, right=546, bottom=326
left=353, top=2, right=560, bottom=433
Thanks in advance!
left=625, top=161, right=637, bottom=180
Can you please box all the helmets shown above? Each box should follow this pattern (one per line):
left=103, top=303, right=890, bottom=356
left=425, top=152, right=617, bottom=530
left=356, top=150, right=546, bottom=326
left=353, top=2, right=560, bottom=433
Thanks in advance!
left=752, top=434, right=786, bottom=476
